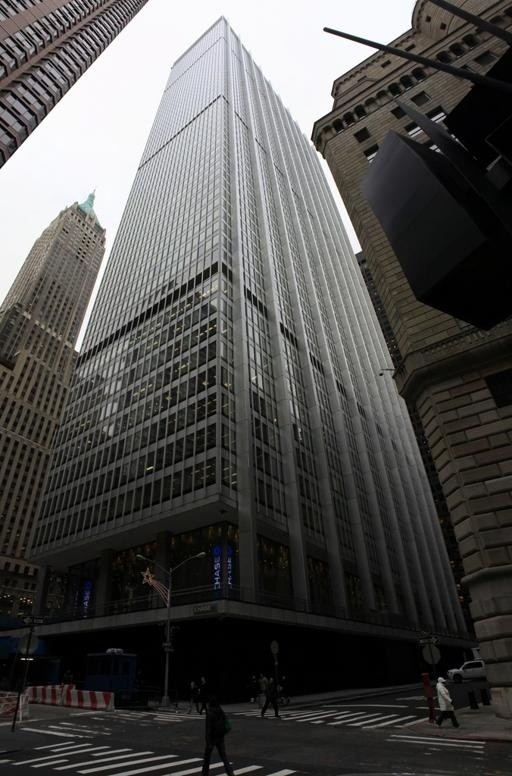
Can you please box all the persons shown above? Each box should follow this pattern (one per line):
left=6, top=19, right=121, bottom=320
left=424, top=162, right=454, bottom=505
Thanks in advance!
left=201, top=696, right=234, bottom=776
left=188, top=676, right=210, bottom=714
left=247, top=672, right=291, bottom=718
left=434, top=676, right=458, bottom=729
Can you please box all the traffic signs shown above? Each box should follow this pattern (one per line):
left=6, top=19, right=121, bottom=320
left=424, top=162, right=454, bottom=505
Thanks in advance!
left=21, top=615, right=45, bottom=626
left=418, top=636, right=440, bottom=645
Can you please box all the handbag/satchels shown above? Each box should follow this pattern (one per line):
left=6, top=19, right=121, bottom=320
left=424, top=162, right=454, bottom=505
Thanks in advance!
left=224, top=719, right=232, bottom=734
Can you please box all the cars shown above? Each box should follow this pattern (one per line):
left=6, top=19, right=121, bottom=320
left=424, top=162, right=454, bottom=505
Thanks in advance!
left=447, top=660, right=486, bottom=682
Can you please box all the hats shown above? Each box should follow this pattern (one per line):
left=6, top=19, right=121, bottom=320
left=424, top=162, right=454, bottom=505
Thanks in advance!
left=438, top=676, right=447, bottom=682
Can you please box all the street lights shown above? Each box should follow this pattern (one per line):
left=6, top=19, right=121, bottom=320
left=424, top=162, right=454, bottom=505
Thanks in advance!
left=136, top=552, right=208, bottom=707
